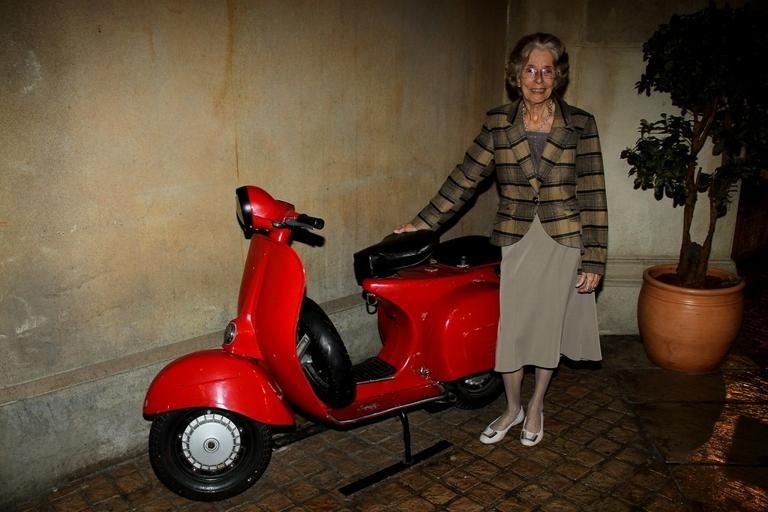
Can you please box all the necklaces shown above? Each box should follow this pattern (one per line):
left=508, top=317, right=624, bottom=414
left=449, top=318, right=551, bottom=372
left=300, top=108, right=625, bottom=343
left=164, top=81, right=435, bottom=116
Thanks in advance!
left=522, top=99, right=553, bottom=131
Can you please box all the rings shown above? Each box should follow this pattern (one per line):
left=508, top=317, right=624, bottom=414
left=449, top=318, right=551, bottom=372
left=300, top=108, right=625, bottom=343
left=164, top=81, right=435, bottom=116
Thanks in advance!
left=590, top=288, right=595, bottom=291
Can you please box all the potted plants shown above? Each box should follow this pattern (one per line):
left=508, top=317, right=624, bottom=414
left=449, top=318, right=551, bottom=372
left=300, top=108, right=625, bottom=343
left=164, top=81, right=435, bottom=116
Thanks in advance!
left=620, top=0, right=768, bottom=376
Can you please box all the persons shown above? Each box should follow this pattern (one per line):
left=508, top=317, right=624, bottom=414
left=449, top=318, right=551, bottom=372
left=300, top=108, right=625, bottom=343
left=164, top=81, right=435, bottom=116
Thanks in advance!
left=393, top=32, right=610, bottom=447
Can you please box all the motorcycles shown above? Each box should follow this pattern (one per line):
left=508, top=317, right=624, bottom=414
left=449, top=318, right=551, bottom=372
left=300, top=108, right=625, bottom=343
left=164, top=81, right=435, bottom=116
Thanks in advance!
left=138, top=181, right=506, bottom=502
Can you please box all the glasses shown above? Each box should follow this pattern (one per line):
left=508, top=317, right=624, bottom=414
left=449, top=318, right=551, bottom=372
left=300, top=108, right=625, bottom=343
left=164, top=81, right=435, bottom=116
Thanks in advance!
left=522, top=66, right=555, bottom=79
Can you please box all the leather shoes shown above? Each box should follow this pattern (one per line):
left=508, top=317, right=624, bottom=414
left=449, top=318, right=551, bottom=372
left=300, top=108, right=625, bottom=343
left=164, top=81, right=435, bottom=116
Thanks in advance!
left=519, top=411, right=544, bottom=448
left=478, top=404, right=526, bottom=446
